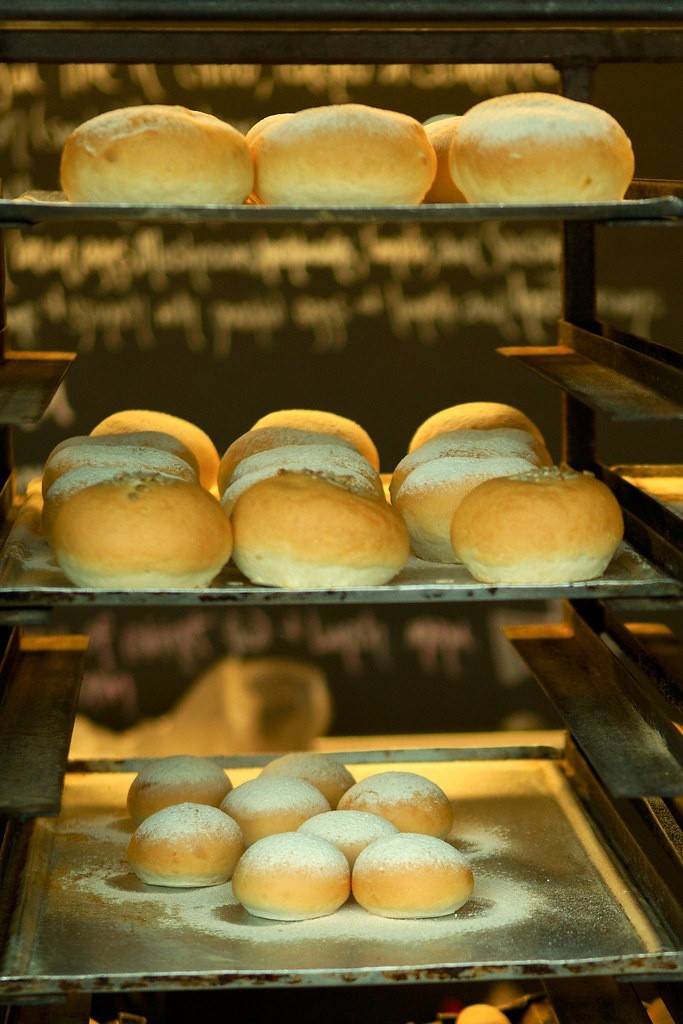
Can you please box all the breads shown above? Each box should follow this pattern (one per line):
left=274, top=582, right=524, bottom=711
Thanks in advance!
left=40, top=408, right=625, bottom=591
left=128, top=752, right=474, bottom=919
left=59, top=91, right=634, bottom=204
